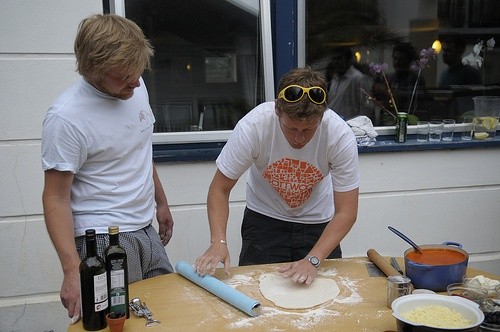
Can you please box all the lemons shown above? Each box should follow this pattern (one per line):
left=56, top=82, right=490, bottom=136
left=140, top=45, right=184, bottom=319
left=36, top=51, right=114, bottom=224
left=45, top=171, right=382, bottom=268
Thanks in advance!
left=474, top=132, right=489, bottom=140
left=481, top=117, right=496, bottom=129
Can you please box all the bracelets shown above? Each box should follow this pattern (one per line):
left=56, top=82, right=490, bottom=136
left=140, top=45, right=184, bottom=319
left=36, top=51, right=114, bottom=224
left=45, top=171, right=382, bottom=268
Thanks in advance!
left=210, top=240, right=227, bottom=245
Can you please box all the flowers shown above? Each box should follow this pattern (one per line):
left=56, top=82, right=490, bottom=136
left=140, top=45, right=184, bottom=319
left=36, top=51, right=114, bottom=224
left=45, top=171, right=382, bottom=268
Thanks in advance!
left=360, top=48, right=438, bottom=118
left=463, top=38, right=496, bottom=88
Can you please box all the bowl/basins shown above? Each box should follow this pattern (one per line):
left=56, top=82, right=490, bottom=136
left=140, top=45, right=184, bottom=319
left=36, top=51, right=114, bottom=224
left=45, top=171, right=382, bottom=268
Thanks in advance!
left=404, top=240, right=469, bottom=291
left=447, top=275, right=500, bottom=331
left=390, top=294, right=485, bottom=332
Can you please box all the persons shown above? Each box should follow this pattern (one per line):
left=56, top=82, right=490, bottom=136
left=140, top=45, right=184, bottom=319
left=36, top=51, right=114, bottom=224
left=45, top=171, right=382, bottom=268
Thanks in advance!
left=41, top=14, right=174, bottom=324
left=195, top=66, right=359, bottom=285
left=371, top=44, right=439, bottom=122
left=310, top=39, right=376, bottom=122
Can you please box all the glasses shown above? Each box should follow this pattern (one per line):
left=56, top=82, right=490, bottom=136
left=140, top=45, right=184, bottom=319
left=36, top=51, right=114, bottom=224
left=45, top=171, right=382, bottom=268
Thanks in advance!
left=277, top=85, right=327, bottom=105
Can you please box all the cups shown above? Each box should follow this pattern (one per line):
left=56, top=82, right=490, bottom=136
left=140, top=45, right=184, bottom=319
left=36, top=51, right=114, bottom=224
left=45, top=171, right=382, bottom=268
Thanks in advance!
left=412, top=289, right=436, bottom=294
left=387, top=275, right=414, bottom=309
left=416, top=116, right=487, bottom=142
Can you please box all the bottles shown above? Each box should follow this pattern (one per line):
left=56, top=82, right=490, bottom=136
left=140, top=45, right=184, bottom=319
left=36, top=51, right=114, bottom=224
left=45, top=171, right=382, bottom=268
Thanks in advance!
left=78, top=229, right=109, bottom=330
left=101, top=226, right=130, bottom=318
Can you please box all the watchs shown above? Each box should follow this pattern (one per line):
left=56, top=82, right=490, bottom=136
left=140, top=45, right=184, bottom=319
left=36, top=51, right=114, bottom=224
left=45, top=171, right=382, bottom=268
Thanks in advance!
left=304, top=254, right=321, bottom=270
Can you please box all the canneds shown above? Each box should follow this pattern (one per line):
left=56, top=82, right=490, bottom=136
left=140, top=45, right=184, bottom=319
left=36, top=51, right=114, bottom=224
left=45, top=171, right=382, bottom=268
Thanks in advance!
left=396, top=112, right=407, bottom=143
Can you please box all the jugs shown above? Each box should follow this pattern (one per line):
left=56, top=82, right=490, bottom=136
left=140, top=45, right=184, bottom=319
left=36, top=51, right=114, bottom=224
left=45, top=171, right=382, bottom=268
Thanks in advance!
left=472, top=96, right=500, bottom=139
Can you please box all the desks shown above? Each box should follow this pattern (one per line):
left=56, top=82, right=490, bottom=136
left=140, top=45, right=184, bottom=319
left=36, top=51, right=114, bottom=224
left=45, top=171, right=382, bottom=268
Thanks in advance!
left=67, top=256, right=500, bottom=332
